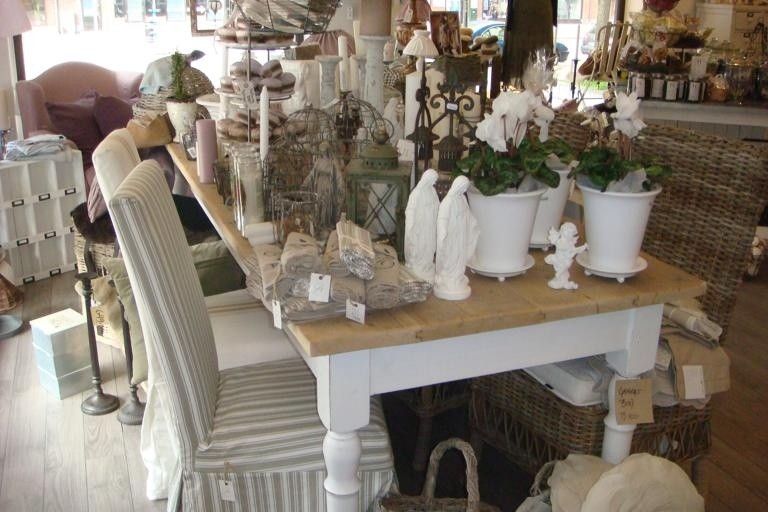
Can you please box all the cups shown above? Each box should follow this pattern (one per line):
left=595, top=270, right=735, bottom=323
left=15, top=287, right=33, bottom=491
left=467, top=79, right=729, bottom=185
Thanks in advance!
left=279, top=190, right=320, bottom=248
left=182, top=133, right=196, bottom=162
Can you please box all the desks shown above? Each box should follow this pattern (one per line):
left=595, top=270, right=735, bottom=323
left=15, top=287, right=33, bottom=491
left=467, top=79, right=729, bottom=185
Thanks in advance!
left=636, top=101, right=768, bottom=142
left=165, top=142, right=708, bottom=512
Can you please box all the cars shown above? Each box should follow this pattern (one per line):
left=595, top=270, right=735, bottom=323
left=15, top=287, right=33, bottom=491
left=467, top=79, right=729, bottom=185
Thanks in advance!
left=471, top=20, right=569, bottom=65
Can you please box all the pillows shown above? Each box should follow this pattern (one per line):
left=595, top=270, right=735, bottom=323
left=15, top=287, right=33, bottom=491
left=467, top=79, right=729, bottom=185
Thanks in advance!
left=92, top=90, right=138, bottom=138
left=44, top=88, right=99, bottom=166
left=87, top=166, right=106, bottom=224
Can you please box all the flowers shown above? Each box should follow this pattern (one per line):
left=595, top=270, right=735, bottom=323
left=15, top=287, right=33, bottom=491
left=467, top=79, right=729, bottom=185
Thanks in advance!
left=447, top=89, right=562, bottom=196
left=566, top=90, right=672, bottom=192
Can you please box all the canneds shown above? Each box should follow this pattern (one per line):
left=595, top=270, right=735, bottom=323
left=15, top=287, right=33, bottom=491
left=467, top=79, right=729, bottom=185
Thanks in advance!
left=626, top=69, right=707, bottom=102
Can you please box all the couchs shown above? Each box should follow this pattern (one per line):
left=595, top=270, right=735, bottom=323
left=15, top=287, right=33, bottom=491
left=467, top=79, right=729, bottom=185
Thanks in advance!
left=16, top=61, right=145, bottom=139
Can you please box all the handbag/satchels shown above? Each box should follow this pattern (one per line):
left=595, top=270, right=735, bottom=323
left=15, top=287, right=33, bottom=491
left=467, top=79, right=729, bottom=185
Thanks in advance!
left=70, top=202, right=120, bottom=241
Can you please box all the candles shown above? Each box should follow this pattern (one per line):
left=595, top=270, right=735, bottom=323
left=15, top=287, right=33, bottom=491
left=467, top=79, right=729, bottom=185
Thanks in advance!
left=337, top=36, right=350, bottom=93
left=259, top=83, right=271, bottom=158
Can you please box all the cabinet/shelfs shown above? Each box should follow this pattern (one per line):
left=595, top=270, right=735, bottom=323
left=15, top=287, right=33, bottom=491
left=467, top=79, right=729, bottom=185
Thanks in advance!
left=216, top=28, right=298, bottom=125
left=0, top=149, right=87, bottom=287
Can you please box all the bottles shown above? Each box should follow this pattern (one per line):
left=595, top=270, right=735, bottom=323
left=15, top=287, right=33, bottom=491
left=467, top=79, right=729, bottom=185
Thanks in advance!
left=228, top=142, right=267, bottom=239
left=196, top=120, right=218, bottom=183
left=627, top=48, right=768, bottom=104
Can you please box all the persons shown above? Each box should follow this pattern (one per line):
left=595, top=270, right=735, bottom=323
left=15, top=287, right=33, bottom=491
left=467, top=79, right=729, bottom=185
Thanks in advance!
left=544, top=221, right=585, bottom=290
left=432, top=175, right=480, bottom=301
left=404, top=168, right=439, bottom=285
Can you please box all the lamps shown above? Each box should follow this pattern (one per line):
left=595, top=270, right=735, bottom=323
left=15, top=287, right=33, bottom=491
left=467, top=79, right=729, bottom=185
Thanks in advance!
left=0, top=0, right=32, bottom=140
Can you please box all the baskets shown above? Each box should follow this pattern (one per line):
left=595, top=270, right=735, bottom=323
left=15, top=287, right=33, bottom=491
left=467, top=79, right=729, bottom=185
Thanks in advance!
left=375, top=374, right=712, bottom=512
left=73, top=227, right=123, bottom=346
left=0, top=273, right=24, bottom=314
left=132, top=67, right=214, bottom=142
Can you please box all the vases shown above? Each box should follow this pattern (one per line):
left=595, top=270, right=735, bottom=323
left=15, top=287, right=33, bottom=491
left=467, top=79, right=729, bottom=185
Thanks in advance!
left=466, top=181, right=548, bottom=282
left=574, top=178, right=664, bottom=283
left=530, top=159, right=577, bottom=253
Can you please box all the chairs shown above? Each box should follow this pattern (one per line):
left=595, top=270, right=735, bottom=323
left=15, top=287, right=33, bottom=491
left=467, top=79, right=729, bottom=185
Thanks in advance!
left=91, top=127, right=301, bottom=502
left=466, top=122, right=768, bottom=493
left=391, top=98, right=590, bottom=473
left=577, top=19, right=636, bottom=107
left=108, top=159, right=400, bottom=512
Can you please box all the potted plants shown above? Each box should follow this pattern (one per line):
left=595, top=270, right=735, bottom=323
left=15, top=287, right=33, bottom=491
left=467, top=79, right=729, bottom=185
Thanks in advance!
left=163, top=50, right=198, bottom=145
